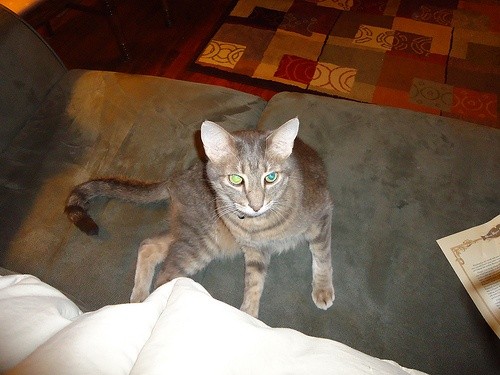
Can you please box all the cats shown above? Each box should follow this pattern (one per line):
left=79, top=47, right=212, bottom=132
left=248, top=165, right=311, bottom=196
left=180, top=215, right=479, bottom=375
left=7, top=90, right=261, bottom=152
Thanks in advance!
left=64, top=115, right=335, bottom=318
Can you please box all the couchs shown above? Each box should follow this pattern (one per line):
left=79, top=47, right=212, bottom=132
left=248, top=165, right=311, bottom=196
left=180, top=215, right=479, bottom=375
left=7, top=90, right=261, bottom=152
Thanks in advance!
left=0, top=5, right=500, bottom=375
